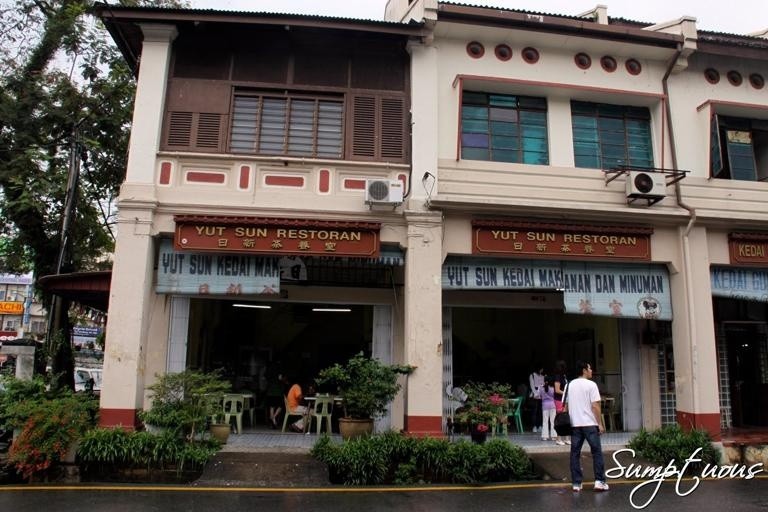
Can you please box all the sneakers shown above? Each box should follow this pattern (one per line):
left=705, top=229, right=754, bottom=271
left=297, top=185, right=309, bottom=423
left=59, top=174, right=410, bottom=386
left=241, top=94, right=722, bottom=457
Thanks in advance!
left=542, top=438, right=547, bottom=441
left=552, top=436, right=561, bottom=441
left=289, top=424, right=293, bottom=432
left=537, top=426, right=542, bottom=433
left=292, top=423, right=303, bottom=433
left=571, top=483, right=583, bottom=491
left=532, top=426, right=538, bottom=433
left=592, top=480, right=610, bottom=491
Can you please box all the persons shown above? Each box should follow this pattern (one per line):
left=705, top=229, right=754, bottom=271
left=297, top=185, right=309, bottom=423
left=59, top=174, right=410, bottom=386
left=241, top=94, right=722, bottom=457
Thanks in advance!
left=287, top=384, right=314, bottom=433
left=529, top=367, right=545, bottom=432
left=554, top=369, right=572, bottom=445
left=267, top=361, right=287, bottom=428
left=540, top=376, right=557, bottom=441
left=561, top=359, right=609, bottom=490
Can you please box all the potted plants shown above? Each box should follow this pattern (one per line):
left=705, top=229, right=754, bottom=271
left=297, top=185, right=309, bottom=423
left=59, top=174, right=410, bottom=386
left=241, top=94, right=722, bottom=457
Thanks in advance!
left=454, top=379, right=515, bottom=443
left=314, top=350, right=418, bottom=441
left=210, top=387, right=232, bottom=445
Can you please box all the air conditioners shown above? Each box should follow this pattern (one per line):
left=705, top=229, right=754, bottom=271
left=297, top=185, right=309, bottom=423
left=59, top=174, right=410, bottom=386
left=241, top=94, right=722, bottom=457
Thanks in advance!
left=364, top=176, right=404, bottom=206
left=624, top=170, right=668, bottom=198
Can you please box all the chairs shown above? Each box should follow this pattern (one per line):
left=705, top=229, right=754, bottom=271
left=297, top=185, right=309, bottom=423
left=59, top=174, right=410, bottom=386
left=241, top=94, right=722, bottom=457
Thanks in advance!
left=307, top=395, right=334, bottom=434
left=281, top=394, right=307, bottom=433
left=497, top=396, right=524, bottom=433
left=220, top=394, right=244, bottom=434
left=239, top=391, right=257, bottom=427
left=601, top=397, right=616, bottom=433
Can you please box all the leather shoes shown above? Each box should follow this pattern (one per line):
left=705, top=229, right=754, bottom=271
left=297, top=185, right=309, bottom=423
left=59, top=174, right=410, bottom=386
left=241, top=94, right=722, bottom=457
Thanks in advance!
left=563, top=440, right=572, bottom=446
left=555, top=440, right=566, bottom=446
